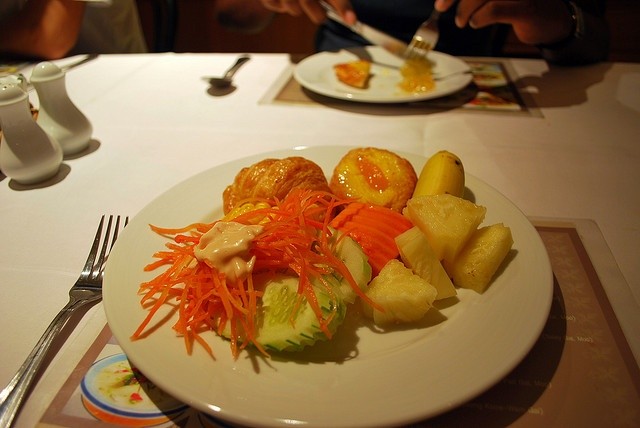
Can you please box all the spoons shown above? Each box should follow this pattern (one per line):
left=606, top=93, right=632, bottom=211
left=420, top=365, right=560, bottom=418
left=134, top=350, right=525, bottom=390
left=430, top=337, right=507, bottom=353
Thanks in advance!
left=200, top=57, right=252, bottom=87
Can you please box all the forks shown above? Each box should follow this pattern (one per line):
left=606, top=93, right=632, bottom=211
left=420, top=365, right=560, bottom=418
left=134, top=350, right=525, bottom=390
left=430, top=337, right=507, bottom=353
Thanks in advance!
left=405, top=2, right=439, bottom=61
left=0, top=213, right=128, bottom=428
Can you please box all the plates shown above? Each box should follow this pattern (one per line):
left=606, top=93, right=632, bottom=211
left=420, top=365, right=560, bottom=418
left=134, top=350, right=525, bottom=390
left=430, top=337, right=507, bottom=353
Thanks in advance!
left=293, top=44, right=473, bottom=103
left=102, top=144, right=554, bottom=428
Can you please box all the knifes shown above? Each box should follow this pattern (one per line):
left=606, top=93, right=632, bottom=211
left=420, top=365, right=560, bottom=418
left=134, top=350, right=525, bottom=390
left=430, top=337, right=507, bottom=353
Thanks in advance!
left=317, top=1, right=435, bottom=67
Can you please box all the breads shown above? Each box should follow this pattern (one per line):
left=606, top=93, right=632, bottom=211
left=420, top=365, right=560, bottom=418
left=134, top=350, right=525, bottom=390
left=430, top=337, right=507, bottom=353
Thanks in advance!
left=329, top=145, right=417, bottom=215
left=221, top=155, right=327, bottom=216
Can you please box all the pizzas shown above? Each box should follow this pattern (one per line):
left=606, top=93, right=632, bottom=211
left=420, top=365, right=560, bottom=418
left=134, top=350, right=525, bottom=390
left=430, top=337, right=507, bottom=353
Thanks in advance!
left=334, top=60, right=371, bottom=89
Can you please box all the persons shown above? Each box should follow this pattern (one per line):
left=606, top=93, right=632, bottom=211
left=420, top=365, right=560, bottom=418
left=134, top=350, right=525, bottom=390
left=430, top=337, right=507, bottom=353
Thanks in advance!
left=0, top=0, right=85, bottom=60
left=254, top=1, right=610, bottom=56
